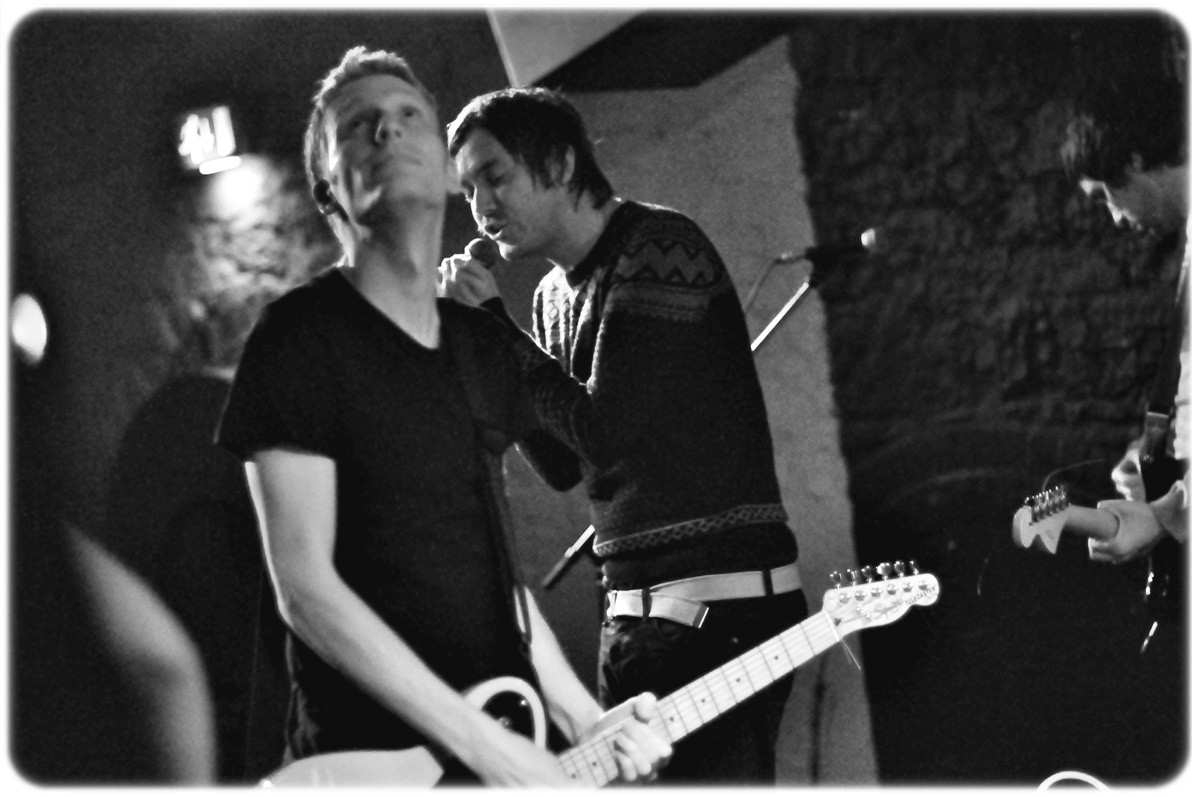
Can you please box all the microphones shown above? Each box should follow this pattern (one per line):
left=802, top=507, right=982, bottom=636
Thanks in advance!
left=465, top=239, right=497, bottom=270
left=777, top=225, right=892, bottom=264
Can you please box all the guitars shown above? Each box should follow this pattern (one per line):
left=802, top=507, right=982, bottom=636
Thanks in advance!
left=1010, top=461, right=1168, bottom=604
left=256, top=561, right=941, bottom=787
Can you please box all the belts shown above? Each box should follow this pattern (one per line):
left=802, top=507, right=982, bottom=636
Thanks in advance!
left=603, top=561, right=802, bottom=630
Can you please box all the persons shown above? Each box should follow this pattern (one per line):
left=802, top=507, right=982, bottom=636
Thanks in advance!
left=1057, top=87, right=1187, bottom=614
left=437, top=90, right=812, bottom=787
left=209, top=44, right=676, bottom=787
